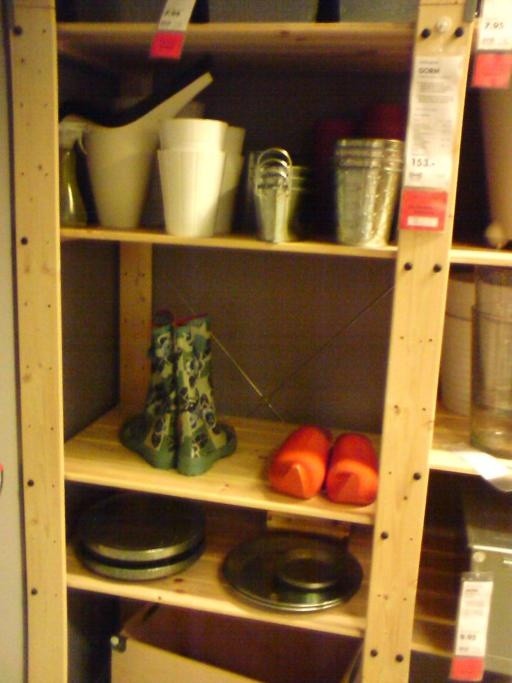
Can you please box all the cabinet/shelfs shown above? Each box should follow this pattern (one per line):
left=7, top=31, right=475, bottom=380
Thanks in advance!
left=0, top=0, right=512, bottom=683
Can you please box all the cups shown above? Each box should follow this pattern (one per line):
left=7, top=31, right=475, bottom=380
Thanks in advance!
left=250, top=147, right=309, bottom=245
left=156, top=118, right=247, bottom=238
left=332, top=138, right=406, bottom=250
left=439, top=270, right=512, bottom=459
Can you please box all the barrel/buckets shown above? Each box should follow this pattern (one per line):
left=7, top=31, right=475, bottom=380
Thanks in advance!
left=252, top=146, right=312, bottom=243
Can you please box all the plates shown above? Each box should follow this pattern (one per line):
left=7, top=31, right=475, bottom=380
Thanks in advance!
left=220, top=534, right=364, bottom=612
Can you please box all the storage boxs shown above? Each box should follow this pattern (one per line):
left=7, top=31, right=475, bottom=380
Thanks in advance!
left=111, top=601, right=364, bottom=681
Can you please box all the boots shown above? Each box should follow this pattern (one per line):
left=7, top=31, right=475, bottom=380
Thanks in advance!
left=119, top=315, right=235, bottom=476
